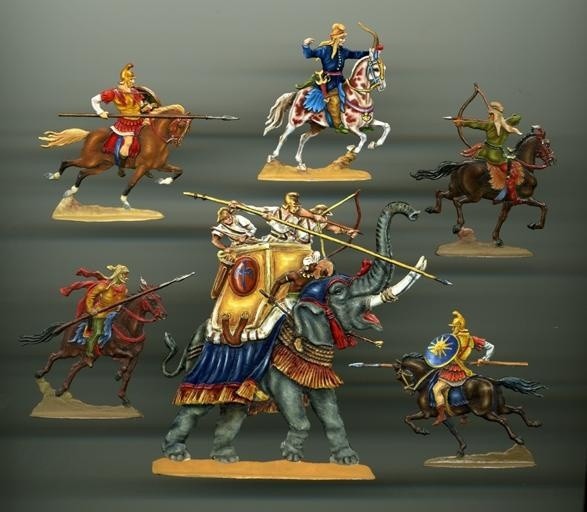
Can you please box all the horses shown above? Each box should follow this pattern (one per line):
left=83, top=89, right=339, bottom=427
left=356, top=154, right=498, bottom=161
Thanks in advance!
left=18, top=276, right=169, bottom=409
left=408, top=125, right=558, bottom=248
left=392, top=352, right=553, bottom=459
left=262, top=47, right=392, bottom=173
left=36, top=104, right=193, bottom=212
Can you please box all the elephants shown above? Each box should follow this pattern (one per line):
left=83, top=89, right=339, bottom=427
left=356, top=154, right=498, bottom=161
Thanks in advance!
left=158, top=201, right=428, bottom=465
